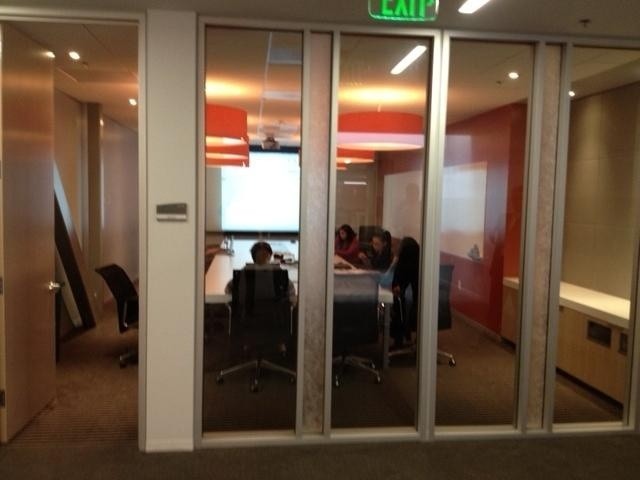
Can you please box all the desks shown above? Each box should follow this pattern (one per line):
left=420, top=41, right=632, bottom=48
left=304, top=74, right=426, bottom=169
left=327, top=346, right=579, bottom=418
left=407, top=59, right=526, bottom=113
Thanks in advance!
left=200, top=234, right=393, bottom=383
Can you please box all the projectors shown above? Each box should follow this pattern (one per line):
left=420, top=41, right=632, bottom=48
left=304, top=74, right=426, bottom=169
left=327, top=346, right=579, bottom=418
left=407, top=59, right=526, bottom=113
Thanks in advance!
left=262, top=140, right=280, bottom=151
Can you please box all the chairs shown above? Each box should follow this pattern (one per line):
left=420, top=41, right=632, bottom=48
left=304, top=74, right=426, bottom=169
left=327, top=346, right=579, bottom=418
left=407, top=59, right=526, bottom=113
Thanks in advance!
left=95, top=260, right=143, bottom=370
left=216, top=268, right=303, bottom=394
left=360, top=225, right=456, bottom=368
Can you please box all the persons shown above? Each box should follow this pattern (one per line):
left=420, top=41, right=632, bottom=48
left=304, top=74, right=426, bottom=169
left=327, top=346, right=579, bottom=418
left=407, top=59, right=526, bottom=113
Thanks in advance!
left=358, top=230, right=391, bottom=268
left=225, top=241, right=298, bottom=361
left=381, top=236, right=420, bottom=350
left=206, top=248, right=231, bottom=256
left=335, top=223, right=359, bottom=256
